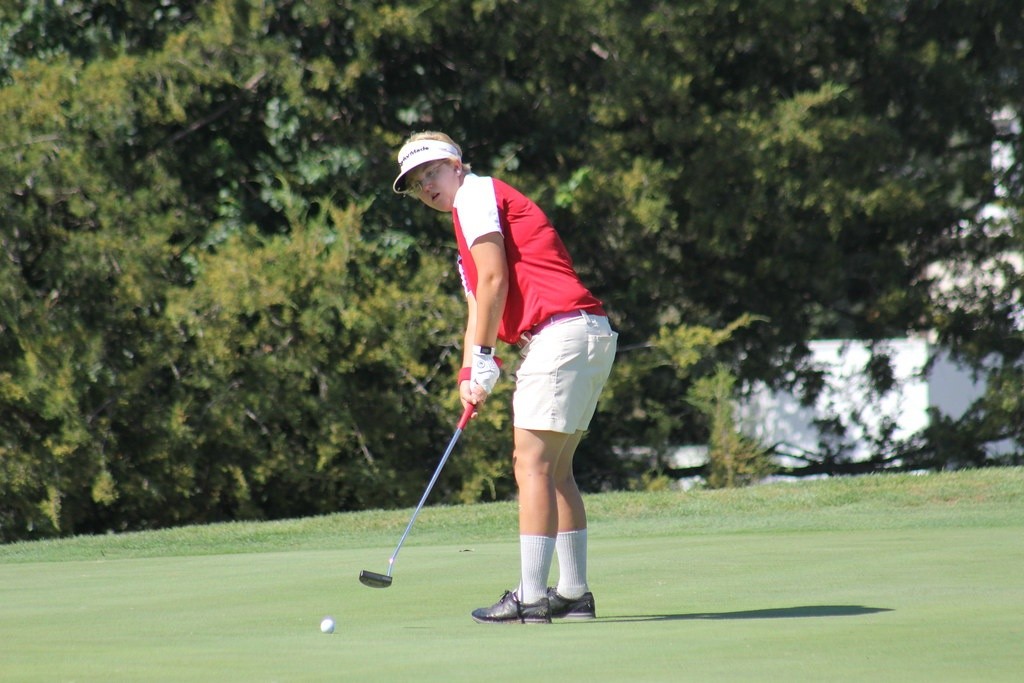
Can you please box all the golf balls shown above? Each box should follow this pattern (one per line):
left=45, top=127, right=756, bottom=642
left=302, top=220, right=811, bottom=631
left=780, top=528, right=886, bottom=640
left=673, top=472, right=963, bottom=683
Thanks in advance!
left=320, top=619, right=335, bottom=634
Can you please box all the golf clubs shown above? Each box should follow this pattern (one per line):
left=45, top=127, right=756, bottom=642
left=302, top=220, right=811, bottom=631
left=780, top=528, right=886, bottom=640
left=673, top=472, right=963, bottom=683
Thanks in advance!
left=358, top=356, right=502, bottom=589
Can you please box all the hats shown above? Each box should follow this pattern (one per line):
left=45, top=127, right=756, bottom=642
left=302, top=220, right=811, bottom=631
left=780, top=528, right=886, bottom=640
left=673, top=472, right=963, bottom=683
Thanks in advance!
left=393, top=138, right=461, bottom=194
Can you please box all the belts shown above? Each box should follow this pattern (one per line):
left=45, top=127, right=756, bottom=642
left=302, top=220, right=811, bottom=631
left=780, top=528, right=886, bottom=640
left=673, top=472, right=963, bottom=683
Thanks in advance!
left=517, top=309, right=592, bottom=348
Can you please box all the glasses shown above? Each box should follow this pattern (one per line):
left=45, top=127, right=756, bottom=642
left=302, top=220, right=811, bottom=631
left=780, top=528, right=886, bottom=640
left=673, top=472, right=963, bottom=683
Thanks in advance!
left=407, top=162, right=448, bottom=194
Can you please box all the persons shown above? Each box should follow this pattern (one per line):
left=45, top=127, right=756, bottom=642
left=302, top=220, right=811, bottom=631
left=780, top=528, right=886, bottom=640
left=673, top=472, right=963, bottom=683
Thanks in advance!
left=392, top=131, right=619, bottom=624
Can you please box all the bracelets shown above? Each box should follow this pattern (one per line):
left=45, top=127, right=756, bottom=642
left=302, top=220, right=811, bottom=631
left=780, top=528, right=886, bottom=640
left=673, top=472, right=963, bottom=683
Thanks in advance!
left=458, top=367, right=471, bottom=385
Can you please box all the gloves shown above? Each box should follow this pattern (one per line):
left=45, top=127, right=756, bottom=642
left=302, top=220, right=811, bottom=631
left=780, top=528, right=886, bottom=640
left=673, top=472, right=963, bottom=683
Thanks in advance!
left=470, top=345, right=500, bottom=396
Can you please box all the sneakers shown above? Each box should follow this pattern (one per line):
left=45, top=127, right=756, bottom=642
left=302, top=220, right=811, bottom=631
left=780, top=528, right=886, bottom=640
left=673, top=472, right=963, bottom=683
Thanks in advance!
left=471, top=590, right=552, bottom=624
left=548, top=587, right=596, bottom=621
